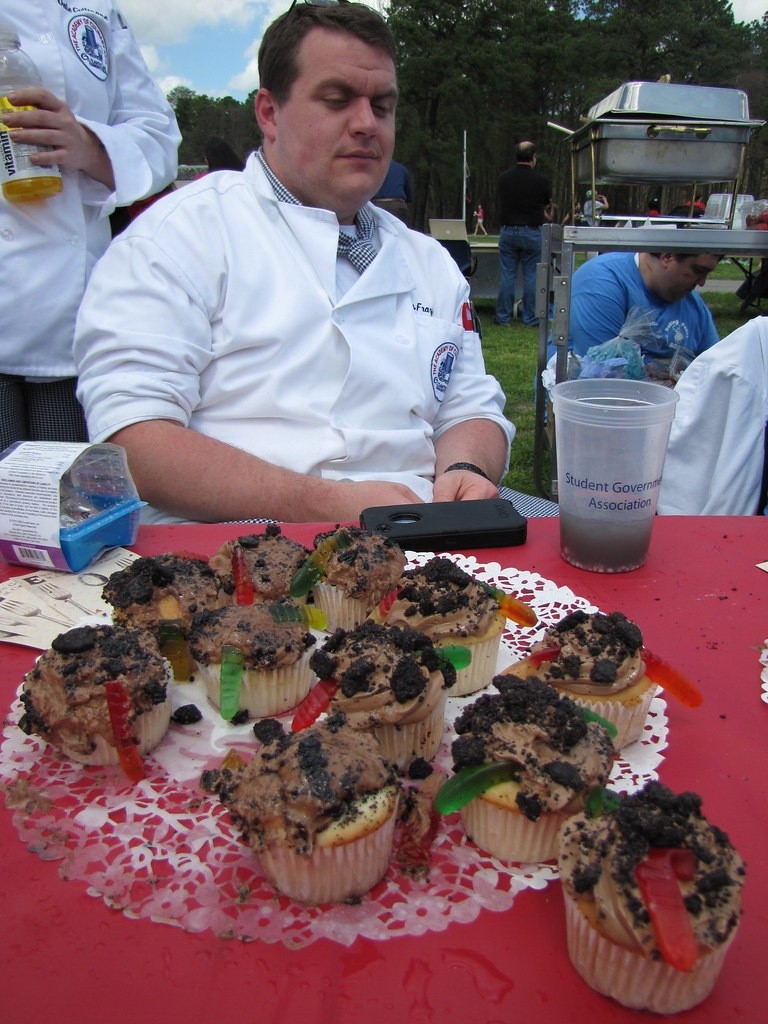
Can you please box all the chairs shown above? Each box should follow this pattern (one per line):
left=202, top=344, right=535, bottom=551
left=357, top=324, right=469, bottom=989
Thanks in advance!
left=437, top=239, right=483, bottom=340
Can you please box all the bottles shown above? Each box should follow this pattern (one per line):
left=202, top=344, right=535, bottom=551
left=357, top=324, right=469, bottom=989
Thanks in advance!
left=0, top=24, right=64, bottom=202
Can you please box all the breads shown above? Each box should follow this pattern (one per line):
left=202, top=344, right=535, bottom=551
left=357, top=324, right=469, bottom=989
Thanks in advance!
left=555, top=780, right=747, bottom=1011
left=21, top=521, right=704, bottom=902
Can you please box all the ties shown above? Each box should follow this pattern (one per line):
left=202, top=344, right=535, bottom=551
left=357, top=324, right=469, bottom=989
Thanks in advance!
left=259, top=145, right=380, bottom=275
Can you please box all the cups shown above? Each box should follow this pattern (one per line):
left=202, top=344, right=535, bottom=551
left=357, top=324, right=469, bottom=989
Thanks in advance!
left=549, top=376, right=681, bottom=573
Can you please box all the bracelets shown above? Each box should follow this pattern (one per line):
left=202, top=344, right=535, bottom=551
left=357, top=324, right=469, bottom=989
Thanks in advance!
left=444, top=462, right=495, bottom=486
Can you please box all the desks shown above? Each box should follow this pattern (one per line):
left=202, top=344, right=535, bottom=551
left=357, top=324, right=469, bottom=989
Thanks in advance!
left=0, top=514, right=768, bottom=1024
left=467, top=235, right=526, bottom=309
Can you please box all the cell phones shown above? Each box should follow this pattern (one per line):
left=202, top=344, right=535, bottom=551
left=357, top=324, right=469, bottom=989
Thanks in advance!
left=360, top=497, right=528, bottom=553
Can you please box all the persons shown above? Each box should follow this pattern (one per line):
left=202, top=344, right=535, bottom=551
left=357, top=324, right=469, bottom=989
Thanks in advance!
left=473, top=204, right=488, bottom=237
left=646, top=196, right=661, bottom=217
left=533, top=205, right=745, bottom=430
left=0, top=0, right=184, bottom=454
left=370, top=158, right=413, bottom=222
left=195, top=136, right=246, bottom=180
left=495, top=139, right=552, bottom=327
left=683, top=191, right=706, bottom=211
left=73, top=0, right=559, bottom=526
left=562, top=190, right=610, bottom=227
left=544, top=198, right=558, bottom=223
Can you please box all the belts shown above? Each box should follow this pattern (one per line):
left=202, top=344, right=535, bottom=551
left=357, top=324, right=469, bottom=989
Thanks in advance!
left=371, top=198, right=404, bottom=201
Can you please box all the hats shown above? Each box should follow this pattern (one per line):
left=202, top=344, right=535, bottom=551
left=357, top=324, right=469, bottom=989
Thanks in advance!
left=586, top=190, right=598, bottom=197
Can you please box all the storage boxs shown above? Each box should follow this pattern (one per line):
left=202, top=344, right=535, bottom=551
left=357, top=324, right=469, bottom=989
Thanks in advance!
left=0, top=441, right=148, bottom=574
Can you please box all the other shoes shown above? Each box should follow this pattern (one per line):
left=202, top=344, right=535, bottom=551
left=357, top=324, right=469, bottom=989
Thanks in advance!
left=496, top=317, right=509, bottom=325
